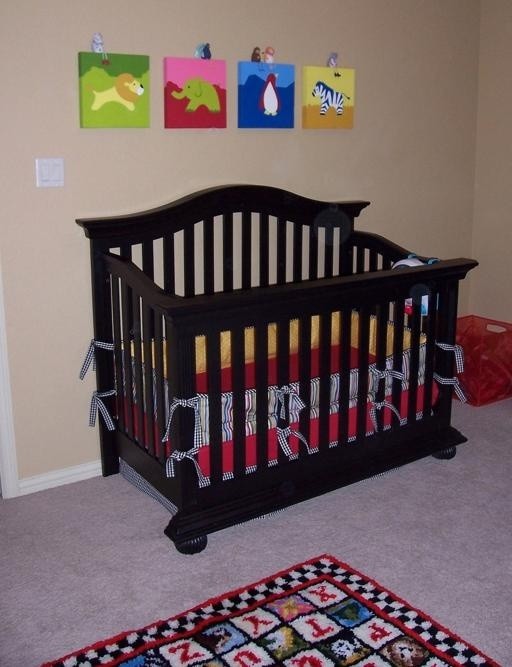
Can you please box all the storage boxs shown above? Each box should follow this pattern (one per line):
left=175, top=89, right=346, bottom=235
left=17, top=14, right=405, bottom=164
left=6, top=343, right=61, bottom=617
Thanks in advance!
left=452, top=315, right=512, bottom=406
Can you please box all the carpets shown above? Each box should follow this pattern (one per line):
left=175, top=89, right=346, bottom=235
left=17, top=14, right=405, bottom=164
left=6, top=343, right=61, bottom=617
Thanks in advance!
left=40, top=553, right=501, bottom=667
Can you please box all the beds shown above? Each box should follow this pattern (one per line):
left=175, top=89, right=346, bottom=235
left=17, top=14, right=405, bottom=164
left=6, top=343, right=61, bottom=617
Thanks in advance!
left=77, top=185, right=478, bottom=553
